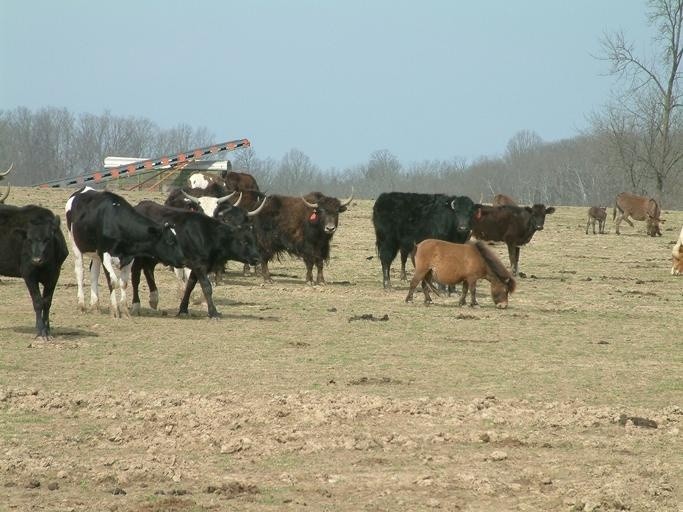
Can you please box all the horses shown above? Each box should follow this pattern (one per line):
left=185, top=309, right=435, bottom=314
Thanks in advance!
left=611, top=193, right=667, bottom=237
left=585, top=204, right=608, bottom=235
left=404, top=237, right=517, bottom=310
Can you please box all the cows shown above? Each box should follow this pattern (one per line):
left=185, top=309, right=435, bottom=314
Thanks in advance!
left=470, top=201, right=556, bottom=274
left=667, top=225, right=683, bottom=276
left=0, top=160, right=68, bottom=343
left=370, top=191, right=483, bottom=289
left=492, top=193, right=517, bottom=206
left=243, top=184, right=357, bottom=288
left=63, top=168, right=268, bottom=322
